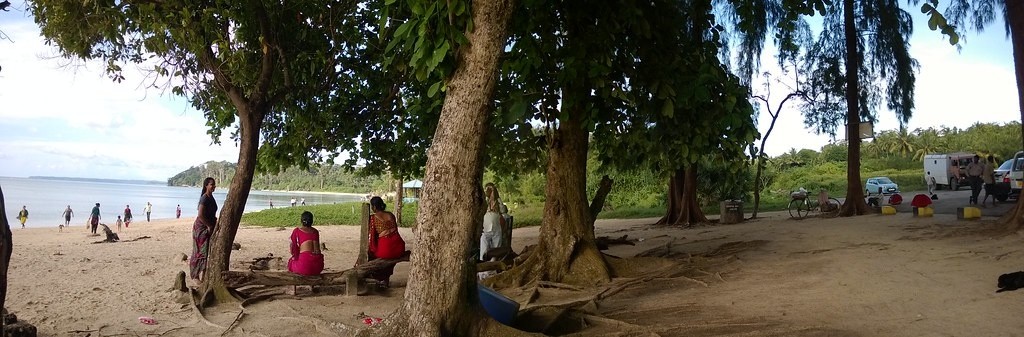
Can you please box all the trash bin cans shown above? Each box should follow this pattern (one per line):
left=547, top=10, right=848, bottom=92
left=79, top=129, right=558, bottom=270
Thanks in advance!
left=720, top=200, right=744, bottom=224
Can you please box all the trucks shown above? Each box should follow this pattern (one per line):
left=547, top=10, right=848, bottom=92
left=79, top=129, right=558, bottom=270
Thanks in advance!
left=923, top=153, right=974, bottom=191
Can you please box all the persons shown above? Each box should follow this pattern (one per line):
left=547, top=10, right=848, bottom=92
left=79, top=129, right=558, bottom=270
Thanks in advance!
left=965, top=155, right=985, bottom=205
left=89, top=203, right=101, bottom=236
left=116, top=216, right=122, bottom=232
left=368, top=196, right=406, bottom=260
left=124, top=205, right=132, bottom=227
left=269, top=197, right=305, bottom=209
left=925, top=171, right=935, bottom=195
left=866, top=187, right=884, bottom=206
left=177, top=204, right=181, bottom=218
left=981, top=155, right=1000, bottom=208
left=62, top=205, right=74, bottom=227
left=145, top=202, right=152, bottom=222
left=17, top=206, right=28, bottom=229
left=189, top=177, right=218, bottom=283
left=480, top=183, right=510, bottom=261
left=287, top=211, right=324, bottom=276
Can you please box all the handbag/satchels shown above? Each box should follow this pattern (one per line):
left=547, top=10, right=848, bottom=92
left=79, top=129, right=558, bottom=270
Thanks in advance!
left=87, top=220, right=90, bottom=229
left=500, top=214, right=505, bottom=226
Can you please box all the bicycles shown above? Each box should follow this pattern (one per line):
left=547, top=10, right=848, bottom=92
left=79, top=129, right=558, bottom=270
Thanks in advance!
left=788, top=188, right=843, bottom=220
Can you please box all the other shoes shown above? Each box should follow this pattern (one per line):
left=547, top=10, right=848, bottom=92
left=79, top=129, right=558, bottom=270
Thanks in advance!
left=981, top=203, right=999, bottom=209
left=353, top=311, right=382, bottom=325
left=970, top=196, right=977, bottom=205
left=139, top=317, right=158, bottom=325
left=376, top=281, right=389, bottom=288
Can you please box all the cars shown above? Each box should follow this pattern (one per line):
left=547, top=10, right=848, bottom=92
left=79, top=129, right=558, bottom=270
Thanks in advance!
left=865, top=177, right=900, bottom=196
left=992, top=158, right=1024, bottom=202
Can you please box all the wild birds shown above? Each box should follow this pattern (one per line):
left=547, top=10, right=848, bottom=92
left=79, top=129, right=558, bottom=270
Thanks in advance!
left=58, top=224, right=64, bottom=232
